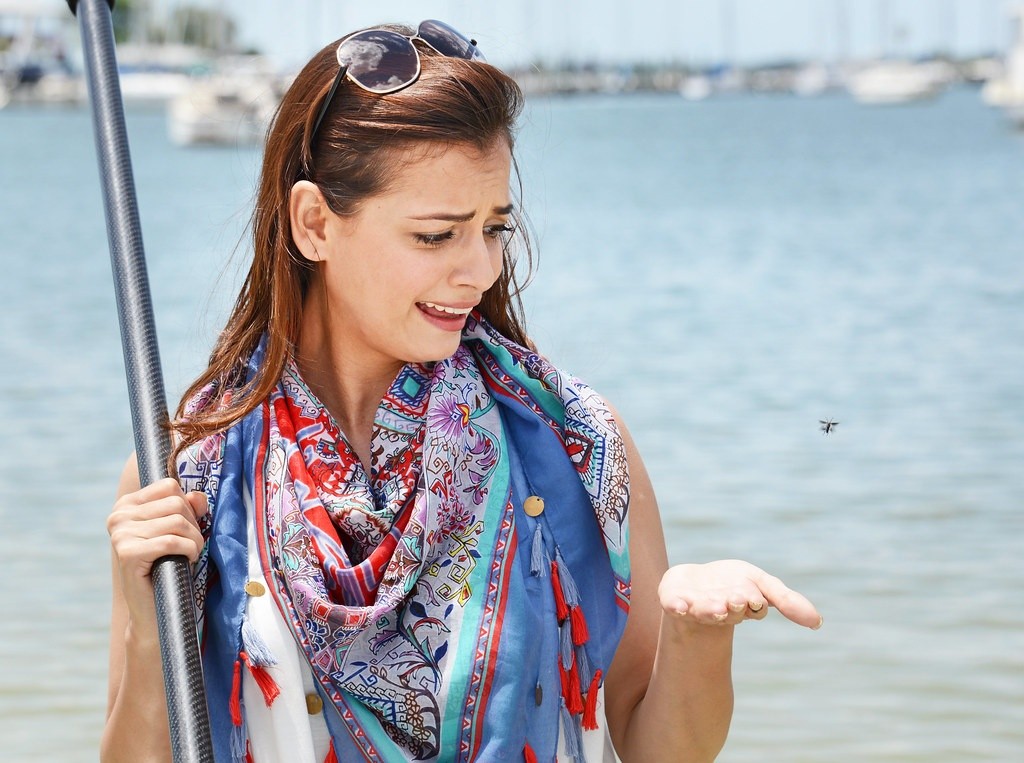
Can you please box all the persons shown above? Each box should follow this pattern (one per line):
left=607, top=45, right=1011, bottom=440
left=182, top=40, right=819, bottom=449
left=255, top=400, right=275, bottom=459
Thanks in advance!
left=99, top=22, right=822, bottom=762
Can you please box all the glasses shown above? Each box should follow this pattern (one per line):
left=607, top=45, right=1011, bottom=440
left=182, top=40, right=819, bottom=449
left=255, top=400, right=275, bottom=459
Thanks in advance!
left=307, top=19, right=489, bottom=149
